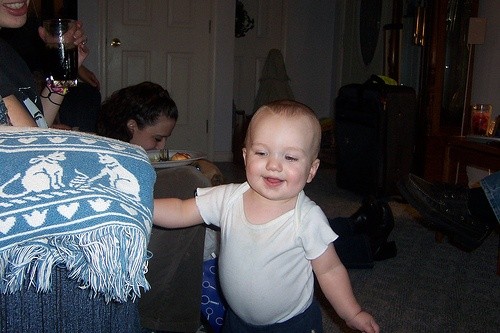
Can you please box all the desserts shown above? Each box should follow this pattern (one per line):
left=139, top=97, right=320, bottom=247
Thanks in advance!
left=171, top=152, right=191, bottom=160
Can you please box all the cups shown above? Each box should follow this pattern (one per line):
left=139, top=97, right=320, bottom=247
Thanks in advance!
left=44, top=18, right=78, bottom=81
left=470, top=103, right=492, bottom=137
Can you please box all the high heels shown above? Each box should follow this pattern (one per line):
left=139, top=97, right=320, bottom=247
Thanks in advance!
left=354, top=202, right=397, bottom=261
left=348, top=200, right=373, bottom=227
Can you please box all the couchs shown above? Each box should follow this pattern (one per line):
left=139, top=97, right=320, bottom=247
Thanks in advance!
left=0, top=129, right=212, bottom=333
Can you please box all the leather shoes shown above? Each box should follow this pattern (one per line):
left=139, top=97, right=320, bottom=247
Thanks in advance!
left=396, top=174, right=492, bottom=251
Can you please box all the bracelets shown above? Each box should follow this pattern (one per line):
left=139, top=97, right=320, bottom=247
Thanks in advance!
left=40, top=85, right=65, bottom=106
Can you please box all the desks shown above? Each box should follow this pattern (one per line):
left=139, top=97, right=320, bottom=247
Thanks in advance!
left=452, top=134, right=500, bottom=186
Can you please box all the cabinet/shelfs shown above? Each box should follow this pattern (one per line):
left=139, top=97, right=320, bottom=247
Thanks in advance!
left=385, top=0, right=479, bottom=185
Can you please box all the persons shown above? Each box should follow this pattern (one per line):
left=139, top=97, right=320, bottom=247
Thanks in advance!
left=0, top=0, right=179, bottom=149
left=396, top=169, right=500, bottom=252
left=152, top=98, right=380, bottom=333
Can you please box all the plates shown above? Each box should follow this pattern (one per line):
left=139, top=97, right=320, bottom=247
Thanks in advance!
left=146, top=149, right=208, bottom=168
